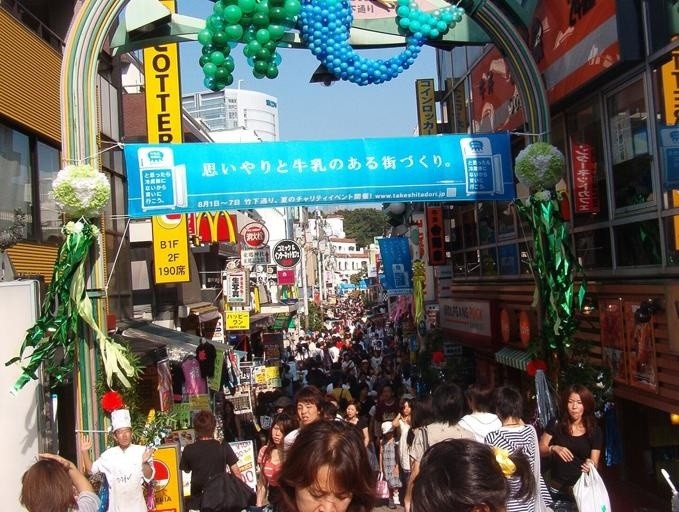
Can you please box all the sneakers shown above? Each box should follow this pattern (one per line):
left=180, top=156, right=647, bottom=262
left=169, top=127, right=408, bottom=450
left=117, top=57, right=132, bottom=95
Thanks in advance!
left=392, top=496, right=399, bottom=504
left=387, top=499, right=396, bottom=509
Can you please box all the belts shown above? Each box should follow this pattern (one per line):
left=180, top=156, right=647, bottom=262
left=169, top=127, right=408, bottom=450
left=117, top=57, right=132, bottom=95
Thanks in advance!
left=550, top=480, right=573, bottom=495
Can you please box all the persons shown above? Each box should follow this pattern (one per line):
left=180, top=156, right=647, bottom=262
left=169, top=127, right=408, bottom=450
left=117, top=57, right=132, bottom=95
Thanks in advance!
left=22, top=283, right=605, bottom=510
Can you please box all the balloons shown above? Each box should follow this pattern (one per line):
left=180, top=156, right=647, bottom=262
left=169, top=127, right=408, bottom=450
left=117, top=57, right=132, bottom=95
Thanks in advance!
left=197, top=0, right=465, bottom=93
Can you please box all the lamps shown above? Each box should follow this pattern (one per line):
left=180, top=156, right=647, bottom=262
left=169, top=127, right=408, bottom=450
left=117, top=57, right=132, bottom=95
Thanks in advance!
left=634, top=300, right=660, bottom=323
left=580, top=299, right=597, bottom=315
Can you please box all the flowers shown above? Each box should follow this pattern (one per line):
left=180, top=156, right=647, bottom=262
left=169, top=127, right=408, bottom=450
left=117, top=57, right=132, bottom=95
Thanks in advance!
left=51, top=164, right=112, bottom=219
left=514, top=142, right=566, bottom=192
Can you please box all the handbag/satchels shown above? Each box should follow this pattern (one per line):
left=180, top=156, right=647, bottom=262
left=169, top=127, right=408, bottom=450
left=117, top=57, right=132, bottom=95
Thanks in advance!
left=375, top=472, right=389, bottom=497
left=200, top=473, right=256, bottom=512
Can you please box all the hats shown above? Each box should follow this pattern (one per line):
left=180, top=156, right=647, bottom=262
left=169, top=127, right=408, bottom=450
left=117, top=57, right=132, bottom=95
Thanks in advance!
left=382, top=422, right=393, bottom=434
left=111, top=409, right=131, bottom=433
